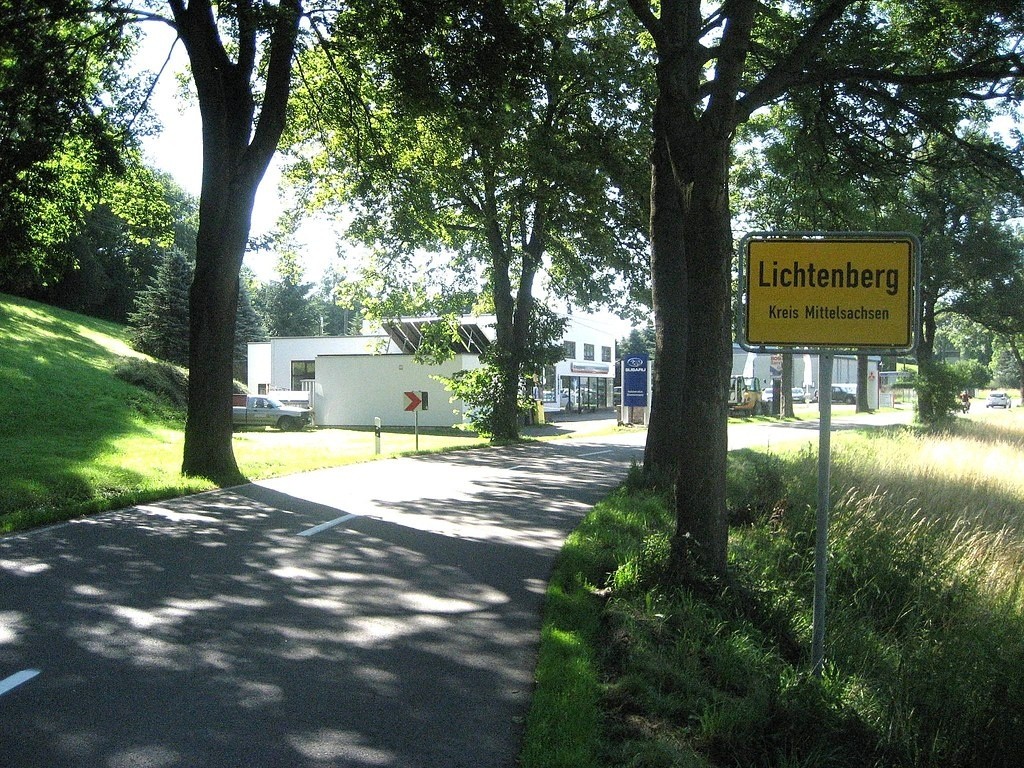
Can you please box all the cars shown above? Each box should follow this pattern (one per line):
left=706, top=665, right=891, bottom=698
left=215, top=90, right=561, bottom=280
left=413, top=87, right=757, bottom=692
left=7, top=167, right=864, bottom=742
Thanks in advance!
left=791, top=387, right=806, bottom=403
left=831, top=385, right=856, bottom=404
left=987, top=392, right=1013, bottom=409
left=762, top=387, right=774, bottom=402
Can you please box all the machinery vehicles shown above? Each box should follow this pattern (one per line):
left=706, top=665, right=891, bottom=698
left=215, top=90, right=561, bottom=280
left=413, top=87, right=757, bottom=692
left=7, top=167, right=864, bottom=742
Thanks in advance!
left=729, top=375, right=768, bottom=418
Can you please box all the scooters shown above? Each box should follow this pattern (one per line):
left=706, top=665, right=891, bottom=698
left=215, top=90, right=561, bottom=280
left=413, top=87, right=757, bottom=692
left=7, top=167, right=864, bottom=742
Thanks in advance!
left=963, top=401, right=971, bottom=414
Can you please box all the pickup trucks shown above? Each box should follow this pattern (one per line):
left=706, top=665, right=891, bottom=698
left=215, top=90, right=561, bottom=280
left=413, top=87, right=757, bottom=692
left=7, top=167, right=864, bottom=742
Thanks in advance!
left=232, top=395, right=311, bottom=431
